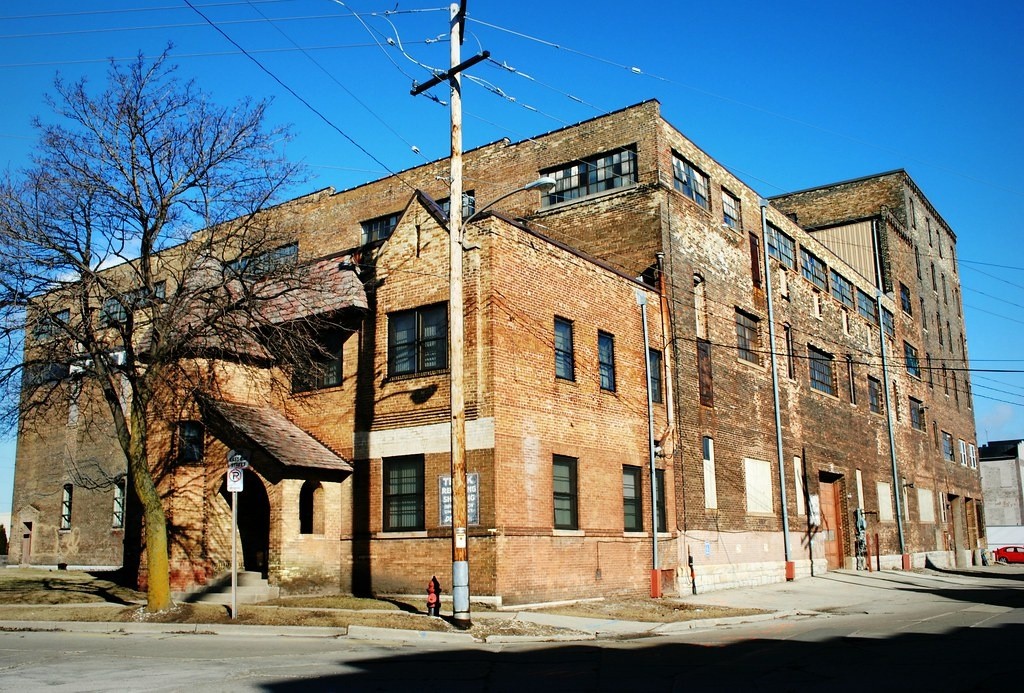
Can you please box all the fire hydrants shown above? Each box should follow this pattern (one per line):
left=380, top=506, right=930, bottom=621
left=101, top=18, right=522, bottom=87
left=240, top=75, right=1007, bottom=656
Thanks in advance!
left=427, top=575, right=443, bottom=617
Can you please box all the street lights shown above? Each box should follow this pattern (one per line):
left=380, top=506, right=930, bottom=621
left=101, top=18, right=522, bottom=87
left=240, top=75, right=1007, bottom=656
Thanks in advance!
left=449, top=176, right=558, bottom=631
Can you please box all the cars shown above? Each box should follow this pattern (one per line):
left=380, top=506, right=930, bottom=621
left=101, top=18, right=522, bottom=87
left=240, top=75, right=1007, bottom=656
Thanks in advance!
left=992, top=545, right=1024, bottom=563
